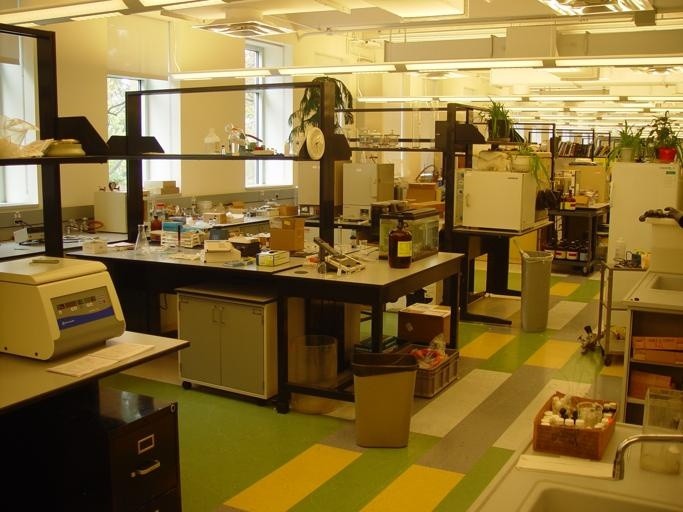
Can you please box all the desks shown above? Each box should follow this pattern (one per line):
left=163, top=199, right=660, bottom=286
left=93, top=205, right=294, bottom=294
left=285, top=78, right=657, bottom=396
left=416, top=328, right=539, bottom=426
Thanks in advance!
left=539, top=203, right=610, bottom=275
left=0, top=330, right=190, bottom=408
left=68, top=242, right=465, bottom=414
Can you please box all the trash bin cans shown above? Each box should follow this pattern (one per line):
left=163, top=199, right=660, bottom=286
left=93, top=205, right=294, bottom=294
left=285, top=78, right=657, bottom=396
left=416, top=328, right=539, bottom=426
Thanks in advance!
left=520, top=250, right=554, bottom=332
left=350, top=352, right=419, bottom=448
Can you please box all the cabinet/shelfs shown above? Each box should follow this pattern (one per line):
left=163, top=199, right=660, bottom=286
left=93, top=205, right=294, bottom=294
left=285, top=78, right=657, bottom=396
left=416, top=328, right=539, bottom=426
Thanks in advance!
left=177, top=287, right=305, bottom=406
left=462, top=170, right=537, bottom=232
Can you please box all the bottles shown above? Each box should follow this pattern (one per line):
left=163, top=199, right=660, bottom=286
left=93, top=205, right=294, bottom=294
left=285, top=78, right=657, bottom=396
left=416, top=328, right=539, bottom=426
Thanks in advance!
left=543, top=238, right=589, bottom=261
left=556, top=189, right=577, bottom=211
left=388, top=215, right=413, bottom=269
left=540, top=395, right=616, bottom=430
left=82, top=218, right=89, bottom=232
left=204, top=124, right=246, bottom=156
left=151, top=215, right=162, bottom=245
left=133, top=224, right=151, bottom=255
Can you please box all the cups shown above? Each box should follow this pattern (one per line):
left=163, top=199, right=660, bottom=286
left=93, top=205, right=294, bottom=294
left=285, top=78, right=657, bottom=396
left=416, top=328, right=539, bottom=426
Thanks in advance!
left=359, top=239, right=367, bottom=249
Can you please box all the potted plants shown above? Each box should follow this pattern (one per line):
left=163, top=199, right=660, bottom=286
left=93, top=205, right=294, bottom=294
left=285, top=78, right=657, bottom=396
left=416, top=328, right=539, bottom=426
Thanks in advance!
left=605, top=111, right=683, bottom=170
left=479, top=100, right=513, bottom=142
left=505, top=138, right=557, bottom=200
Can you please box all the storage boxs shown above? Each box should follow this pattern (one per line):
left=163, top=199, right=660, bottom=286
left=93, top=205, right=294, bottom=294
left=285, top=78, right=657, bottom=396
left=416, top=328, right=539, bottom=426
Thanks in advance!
left=398, top=303, right=460, bottom=346
left=629, top=370, right=676, bottom=401
